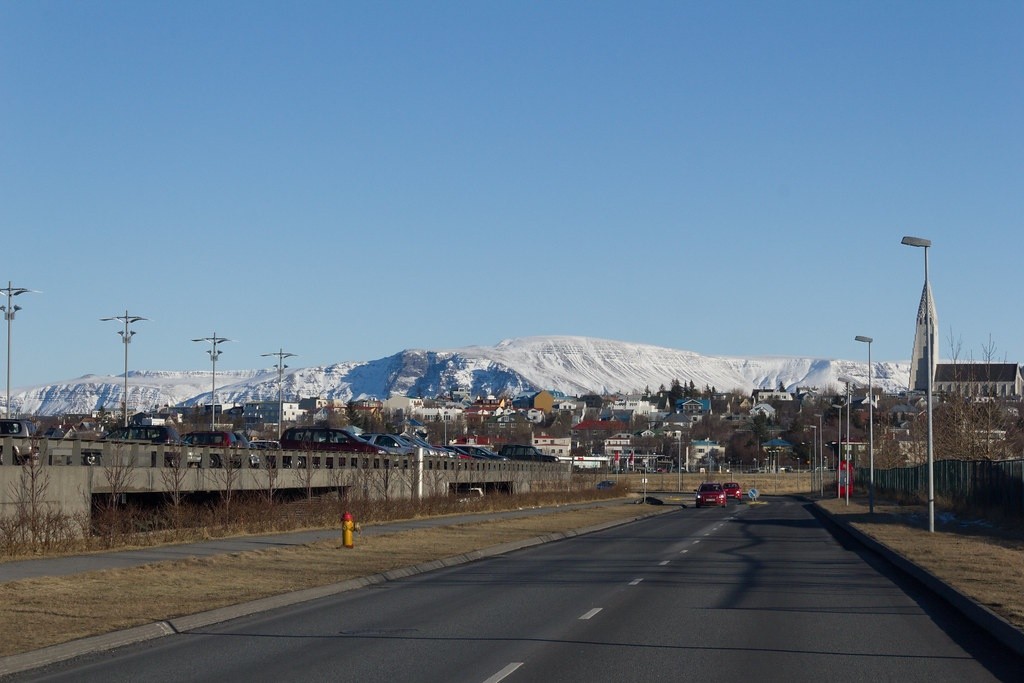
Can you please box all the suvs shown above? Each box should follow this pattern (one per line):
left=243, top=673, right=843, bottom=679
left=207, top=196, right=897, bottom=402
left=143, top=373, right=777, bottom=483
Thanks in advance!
left=280, top=425, right=392, bottom=469
left=0, top=419, right=285, bottom=470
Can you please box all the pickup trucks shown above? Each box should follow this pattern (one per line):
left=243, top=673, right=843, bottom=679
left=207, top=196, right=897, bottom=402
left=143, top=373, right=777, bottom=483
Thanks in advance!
left=493, top=444, right=560, bottom=463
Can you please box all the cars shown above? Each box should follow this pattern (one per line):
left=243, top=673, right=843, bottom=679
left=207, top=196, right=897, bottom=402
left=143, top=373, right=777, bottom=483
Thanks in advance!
left=596, top=480, right=617, bottom=490
left=693, top=482, right=729, bottom=509
left=574, top=461, right=741, bottom=474
left=722, top=482, right=742, bottom=504
left=328, top=429, right=518, bottom=470
left=742, top=465, right=827, bottom=474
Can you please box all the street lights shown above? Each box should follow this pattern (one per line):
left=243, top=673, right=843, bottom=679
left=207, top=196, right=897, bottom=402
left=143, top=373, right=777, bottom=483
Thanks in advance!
left=190, top=331, right=232, bottom=431
left=855, top=336, right=874, bottom=515
left=99, top=310, right=149, bottom=427
left=814, top=414, right=824, bottom=497
left=675, top=430, right=682, bottom=492
left=832, top=403, right=841, bottom=500
left=0, top=279, right=33, bottom=419
left=810, top=425, right=817, bottom=492
left=837, top=377, right=849, bottom=507
left=260, top=347, right=298, bottom=442
left=902, top=236, right=934, bottom=534
